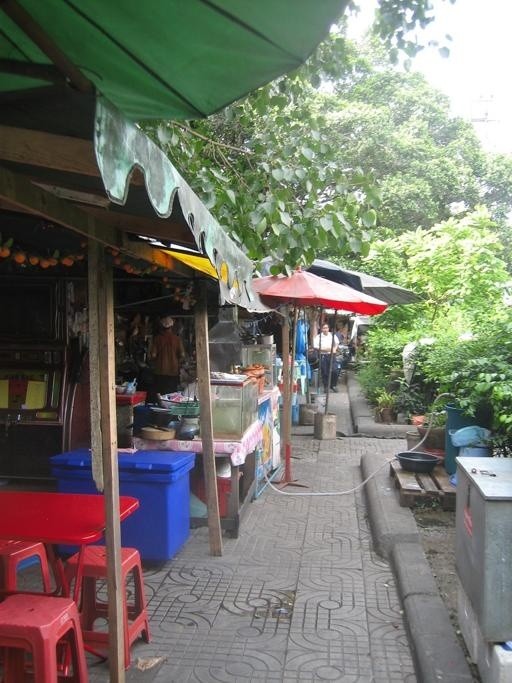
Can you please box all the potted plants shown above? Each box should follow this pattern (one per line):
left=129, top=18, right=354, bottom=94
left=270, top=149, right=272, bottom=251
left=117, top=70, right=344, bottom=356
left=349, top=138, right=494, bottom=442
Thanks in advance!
left=385, top=362, right=405, bottom=381
left=376, top=393, right=395, bottom=423
left=416, top=404, right=447, bottom=449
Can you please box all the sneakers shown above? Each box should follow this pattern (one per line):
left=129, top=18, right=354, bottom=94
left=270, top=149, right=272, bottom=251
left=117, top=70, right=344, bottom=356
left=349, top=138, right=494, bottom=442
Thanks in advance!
left=332, top=386, right=338, bottom=392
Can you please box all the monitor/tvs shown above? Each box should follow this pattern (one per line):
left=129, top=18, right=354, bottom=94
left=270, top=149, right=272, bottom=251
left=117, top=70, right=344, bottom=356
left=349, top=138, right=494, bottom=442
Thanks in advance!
left=0, top=283, right=53, bottom=338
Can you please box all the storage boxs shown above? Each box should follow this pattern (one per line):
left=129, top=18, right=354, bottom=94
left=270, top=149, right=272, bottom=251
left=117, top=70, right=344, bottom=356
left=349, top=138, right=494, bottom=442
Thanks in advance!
left=50, top=447, right=196, bottom=562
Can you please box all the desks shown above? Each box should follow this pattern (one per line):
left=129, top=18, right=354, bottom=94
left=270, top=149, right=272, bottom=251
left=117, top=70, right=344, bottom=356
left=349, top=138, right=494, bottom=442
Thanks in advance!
left=134, top=420, right=262, bottom=539
left=0, top=490, right=140, bottom=680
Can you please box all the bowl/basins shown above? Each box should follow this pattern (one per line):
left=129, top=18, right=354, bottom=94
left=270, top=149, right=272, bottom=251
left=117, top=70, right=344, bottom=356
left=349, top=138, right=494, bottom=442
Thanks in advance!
left=395, top=451, right=440, bottom=473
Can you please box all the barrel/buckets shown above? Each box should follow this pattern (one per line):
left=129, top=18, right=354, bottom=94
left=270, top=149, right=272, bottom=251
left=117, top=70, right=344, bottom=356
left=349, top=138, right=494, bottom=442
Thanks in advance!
left=442, top=403, right=491, bottom=475
left=449, top=440, right=492, bottom=486
left=191, top=460, right=231, bottom=519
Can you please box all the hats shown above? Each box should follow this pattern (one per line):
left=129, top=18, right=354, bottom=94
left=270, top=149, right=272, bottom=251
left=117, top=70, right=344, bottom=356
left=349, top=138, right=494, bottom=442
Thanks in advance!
left=161, top=316, right=176, bottom=328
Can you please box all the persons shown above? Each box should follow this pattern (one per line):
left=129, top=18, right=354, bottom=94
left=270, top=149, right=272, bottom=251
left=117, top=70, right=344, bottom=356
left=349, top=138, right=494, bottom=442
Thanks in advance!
left=147, top=317, right=185, bottom=401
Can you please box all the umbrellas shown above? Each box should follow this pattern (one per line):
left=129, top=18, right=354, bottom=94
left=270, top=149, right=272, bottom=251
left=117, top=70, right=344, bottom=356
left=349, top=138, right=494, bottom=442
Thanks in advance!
left=249, top=257, right=425, bottom=408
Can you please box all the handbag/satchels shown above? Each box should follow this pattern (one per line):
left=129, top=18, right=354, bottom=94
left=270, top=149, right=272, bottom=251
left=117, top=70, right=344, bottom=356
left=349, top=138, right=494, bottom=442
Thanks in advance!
left=308, top=349, right=320, bottom=369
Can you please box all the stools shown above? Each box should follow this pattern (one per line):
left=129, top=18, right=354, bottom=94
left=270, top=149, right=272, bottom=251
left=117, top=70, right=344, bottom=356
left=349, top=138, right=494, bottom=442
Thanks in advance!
left=0, top=539, right=152, bottom=683
